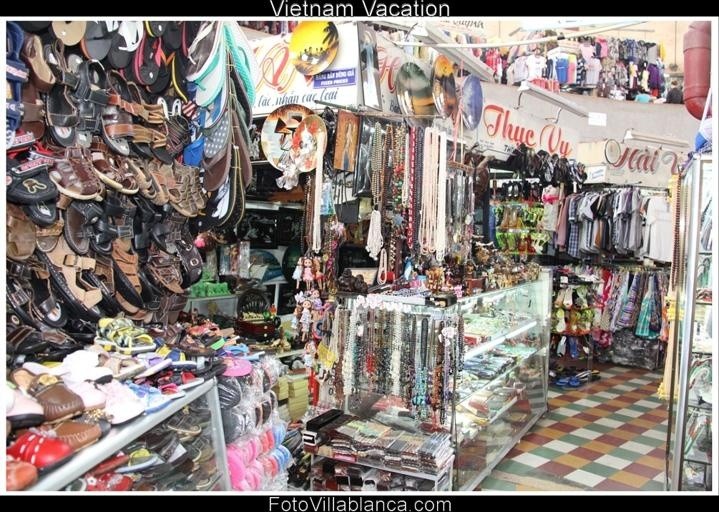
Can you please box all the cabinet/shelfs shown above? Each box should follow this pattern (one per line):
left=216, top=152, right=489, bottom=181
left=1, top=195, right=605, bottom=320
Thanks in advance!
left=665, top=151, right=713, bottom=491
left=313, top=263, right=556, bottom=490
left=24, top=376, right=237, bottom=492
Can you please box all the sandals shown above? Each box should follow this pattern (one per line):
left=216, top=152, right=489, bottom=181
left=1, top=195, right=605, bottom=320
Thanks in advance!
left=549, top=288, right=599, bottom=387
left=7, top=21, right=292, bottom=491
left=500, top=146, right=586, bottom=199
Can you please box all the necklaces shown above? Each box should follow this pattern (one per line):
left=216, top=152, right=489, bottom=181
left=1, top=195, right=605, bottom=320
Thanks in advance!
left=300, top=183, right=312, bottom=255
left=312, top=130, right=324, bottom=254
left=323, top=296, right=465, bottom=424
left=371, top=123, right=447, bottom=262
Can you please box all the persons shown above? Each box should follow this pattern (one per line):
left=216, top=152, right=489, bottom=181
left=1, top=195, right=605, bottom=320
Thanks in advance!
left=292, top=257, right=324, bottom=371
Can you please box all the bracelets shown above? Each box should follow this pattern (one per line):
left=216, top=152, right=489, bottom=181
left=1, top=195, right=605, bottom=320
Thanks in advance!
left=377, top=247, right=388, bottom=285
left=367, top=209, right=382, bottom=259
